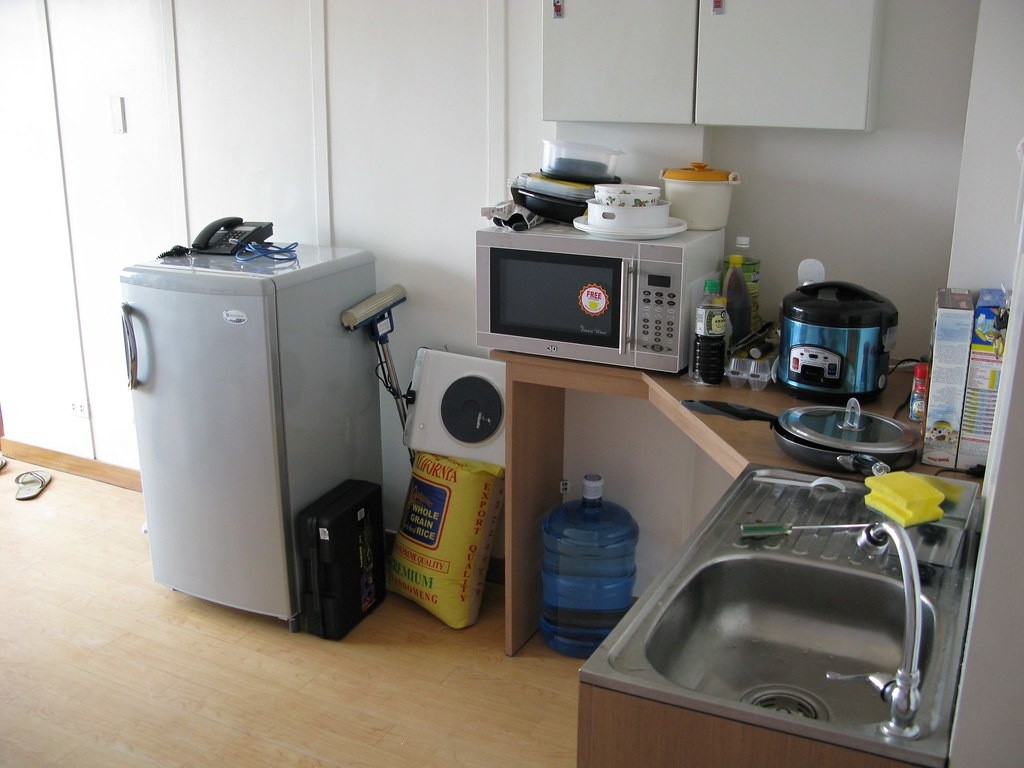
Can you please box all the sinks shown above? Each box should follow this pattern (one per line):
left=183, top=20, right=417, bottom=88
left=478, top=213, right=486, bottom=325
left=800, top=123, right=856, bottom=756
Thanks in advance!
left=574, top=544, right=966, bottom=762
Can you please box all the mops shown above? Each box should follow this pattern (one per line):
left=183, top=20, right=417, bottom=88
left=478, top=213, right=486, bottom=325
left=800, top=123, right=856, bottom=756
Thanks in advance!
left=339, top=282, right=426, bottom=472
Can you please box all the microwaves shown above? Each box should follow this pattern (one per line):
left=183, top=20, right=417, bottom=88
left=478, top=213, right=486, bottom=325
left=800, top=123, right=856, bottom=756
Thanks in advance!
left=475, top=226, right=726, bottom=374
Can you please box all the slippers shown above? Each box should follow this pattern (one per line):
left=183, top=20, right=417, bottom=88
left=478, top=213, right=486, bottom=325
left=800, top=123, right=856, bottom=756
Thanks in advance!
left=0, top=456, right=7, bottom=471
left=14, top=469, right=52, bottom=501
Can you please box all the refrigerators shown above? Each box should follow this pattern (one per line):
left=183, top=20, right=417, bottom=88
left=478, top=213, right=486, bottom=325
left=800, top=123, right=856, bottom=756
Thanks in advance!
left=119, top=241, right=386, bottom=634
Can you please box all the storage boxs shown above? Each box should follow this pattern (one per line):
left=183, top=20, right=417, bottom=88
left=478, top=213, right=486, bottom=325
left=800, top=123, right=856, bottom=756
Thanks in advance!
left=955, top=287, right=1013, bottom=471
left=921, top=289, right=974, bottom=470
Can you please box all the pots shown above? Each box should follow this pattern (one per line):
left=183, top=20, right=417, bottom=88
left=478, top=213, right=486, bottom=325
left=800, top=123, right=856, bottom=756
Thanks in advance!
left=657, top=161, right=741, bottom=231
left=776, top=280, right=899, bottom=407
left=681, top=397, right=924, bottom=477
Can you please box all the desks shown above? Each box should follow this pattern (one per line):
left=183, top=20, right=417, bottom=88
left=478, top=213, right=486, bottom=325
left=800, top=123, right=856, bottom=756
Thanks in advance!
left=488, top=346, right=984, bottom=657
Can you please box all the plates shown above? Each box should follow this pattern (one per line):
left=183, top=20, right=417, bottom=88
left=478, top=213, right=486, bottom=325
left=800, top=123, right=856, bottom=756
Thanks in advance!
left=539, top=169, right=622, bottom=186
left=510, top=186, right=588, bottom=225
left=573, top=215, right=687, bottom=240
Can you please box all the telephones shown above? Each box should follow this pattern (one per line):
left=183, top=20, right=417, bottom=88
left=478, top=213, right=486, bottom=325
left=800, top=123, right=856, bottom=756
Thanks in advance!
left=192, top=216, right=273, bottom=256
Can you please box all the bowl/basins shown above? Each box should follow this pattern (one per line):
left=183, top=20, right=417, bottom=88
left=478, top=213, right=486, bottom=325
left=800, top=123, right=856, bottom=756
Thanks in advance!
left=541, top=139, right=626, bottom=178
left=593, top=183, right=661, bottom=207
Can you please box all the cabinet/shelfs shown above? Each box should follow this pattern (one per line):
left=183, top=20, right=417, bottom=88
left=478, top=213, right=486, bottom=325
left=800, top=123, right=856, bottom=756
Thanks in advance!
left=576, top=677, right=932, bottom=768
left=542, top=0, right=700, bottom=125
left=696, top=0, right=886, bottom=132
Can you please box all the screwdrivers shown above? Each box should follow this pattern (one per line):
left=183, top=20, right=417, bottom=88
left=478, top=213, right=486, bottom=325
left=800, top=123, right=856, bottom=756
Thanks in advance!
left=741, top=521, right=876, bottom=540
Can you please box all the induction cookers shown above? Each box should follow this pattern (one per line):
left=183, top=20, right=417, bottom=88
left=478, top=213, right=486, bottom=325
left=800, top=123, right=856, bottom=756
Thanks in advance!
left=402, top=347, right=506, bottom=468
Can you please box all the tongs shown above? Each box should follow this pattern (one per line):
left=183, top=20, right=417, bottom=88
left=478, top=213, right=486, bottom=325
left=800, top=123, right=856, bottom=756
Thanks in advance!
left=728, top=321, right=774, bottom=358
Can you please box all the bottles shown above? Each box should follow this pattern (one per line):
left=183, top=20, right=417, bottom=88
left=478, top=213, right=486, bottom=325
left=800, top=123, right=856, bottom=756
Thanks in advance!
left=722, top=254, right=753, bottom=343
left=538, top=472, right=640, bottom=660
left=720, top=235, right=761, bottom=332
left=909, top=354, right=930, bottom=423
left=692, top=278, right=727, bottom=387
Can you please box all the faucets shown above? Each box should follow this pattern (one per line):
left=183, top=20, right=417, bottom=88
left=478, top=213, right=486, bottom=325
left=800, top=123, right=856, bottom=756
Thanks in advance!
left=825, top=520, right=930, bottom=739
